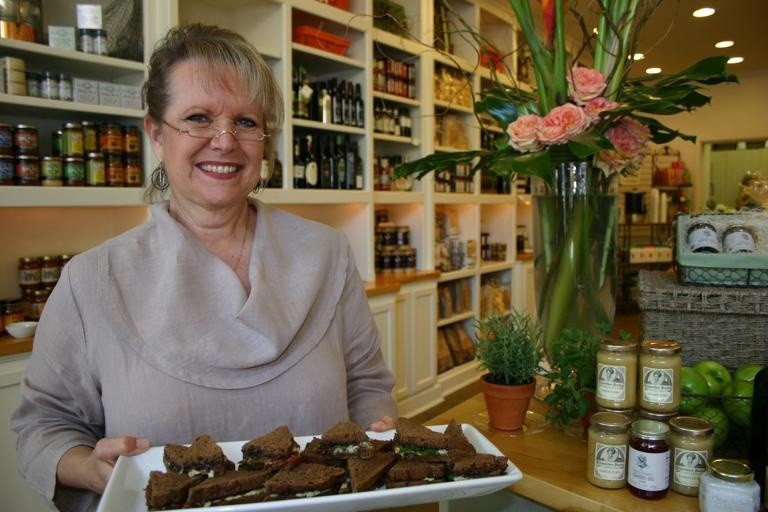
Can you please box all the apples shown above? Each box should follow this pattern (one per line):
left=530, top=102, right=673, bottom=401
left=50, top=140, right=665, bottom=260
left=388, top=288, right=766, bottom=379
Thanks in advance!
left=680, top=360, right=765, bottom=449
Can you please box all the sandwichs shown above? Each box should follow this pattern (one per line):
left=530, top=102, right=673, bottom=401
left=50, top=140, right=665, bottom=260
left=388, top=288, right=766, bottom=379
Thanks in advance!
left=145, top=417, right=509, bottom=512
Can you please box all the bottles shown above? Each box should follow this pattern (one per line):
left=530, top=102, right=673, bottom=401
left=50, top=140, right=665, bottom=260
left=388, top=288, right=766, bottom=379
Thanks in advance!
left=373, top=151, right=392, bottom=191
left=483, top=132, right=495, bottom=151
left=291, top=66, right=367, bottom=191
left=375, top=107, right=410, bottom=136
left=261, top=151, right=283, bottom=189
left=434, top=162, right=473, bottom=193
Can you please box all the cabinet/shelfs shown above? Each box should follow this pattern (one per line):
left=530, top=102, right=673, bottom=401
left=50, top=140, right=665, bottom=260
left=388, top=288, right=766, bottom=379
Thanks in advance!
left=283, top=2, right=373, bottom=203
left=370, top=0, right=427, bottom=203
left=428, top=0, right=577, bottom=206
left=0, top=35, right=154, bottom=207
left=364, top=254, right=535, bottom=424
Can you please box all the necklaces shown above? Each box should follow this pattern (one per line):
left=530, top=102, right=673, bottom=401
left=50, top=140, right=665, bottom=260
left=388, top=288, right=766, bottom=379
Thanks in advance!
left=228, top=201, right=255, bottom=276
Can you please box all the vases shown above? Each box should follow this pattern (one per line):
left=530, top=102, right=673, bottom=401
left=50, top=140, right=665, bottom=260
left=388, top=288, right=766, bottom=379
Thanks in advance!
left=529, top=194, right=623, bottom=396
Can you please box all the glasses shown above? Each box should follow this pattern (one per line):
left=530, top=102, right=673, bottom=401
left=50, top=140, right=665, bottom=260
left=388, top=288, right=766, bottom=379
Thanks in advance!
left=159, top=116, right=272, bottom=142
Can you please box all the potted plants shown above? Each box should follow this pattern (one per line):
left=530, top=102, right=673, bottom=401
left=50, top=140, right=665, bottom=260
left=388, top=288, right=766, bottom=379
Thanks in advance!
left=538, top=325, right=597, bottom=436
left=470, top=306, right=541, bottom=431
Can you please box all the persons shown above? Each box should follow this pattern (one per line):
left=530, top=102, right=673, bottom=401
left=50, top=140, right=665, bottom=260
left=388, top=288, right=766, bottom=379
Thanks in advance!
left=8, top=25, right=401, bottom=510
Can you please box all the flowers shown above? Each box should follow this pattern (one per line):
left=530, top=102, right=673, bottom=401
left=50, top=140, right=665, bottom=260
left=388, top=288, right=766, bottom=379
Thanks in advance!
left=384, top=1, right=739, bottom=366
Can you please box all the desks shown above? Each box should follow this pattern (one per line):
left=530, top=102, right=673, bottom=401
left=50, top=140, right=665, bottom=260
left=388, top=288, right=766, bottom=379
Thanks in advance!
left=420, top=388, right=718, bottom=510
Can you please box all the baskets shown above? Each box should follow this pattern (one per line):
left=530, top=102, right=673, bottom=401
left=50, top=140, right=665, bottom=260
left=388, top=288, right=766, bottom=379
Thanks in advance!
left=672, top=209, right=768, bottom=288
left=680, top=366, right=754, bottom=456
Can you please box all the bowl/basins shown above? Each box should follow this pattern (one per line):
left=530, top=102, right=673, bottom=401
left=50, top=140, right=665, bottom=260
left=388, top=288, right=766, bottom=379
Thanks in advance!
left=4, top=322, right=37, bottom=340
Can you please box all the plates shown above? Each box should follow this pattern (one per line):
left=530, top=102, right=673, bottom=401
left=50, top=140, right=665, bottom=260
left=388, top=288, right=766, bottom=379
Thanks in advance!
left=94, top=421, right=526, bottom=512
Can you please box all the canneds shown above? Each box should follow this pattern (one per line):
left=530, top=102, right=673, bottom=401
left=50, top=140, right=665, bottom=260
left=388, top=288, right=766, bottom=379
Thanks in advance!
left=699, top=457, right=761, bottom=512
left=0, top=118, right=144, bottom=186
left=0, top=253, right=73, bottom=338
left=723, top=223, right=755, bottom=253
left=686, top=220, right=720, bottom=253
left=586, top=339, right=714, bottom=500
